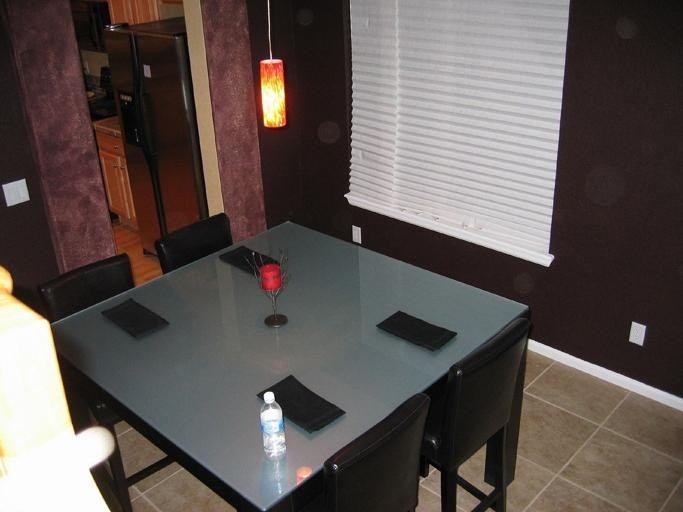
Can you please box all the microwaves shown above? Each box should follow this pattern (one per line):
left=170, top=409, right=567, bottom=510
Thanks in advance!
left=75, top=11, right=110, bottom=52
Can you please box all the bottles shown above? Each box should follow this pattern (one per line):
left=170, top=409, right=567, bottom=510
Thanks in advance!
left=261, top=392, right=286, bottom=464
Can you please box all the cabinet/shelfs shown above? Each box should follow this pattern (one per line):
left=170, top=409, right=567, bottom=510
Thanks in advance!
left=95, top=132, right=138, bottom=230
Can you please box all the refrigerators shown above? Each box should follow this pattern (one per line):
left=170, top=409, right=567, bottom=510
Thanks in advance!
left=104, top=17, right=207, bottom=257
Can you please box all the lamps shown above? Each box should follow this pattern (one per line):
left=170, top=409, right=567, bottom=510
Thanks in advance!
left=258, top=0, right=287, bottom=129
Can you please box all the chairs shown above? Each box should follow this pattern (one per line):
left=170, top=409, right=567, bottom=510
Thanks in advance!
left=40, top=251, right=174, bottom=512
left=153, top=211, right=234, bottom=275
left=420, top=318, right=531, bottom=512
left=297, top=392, right=430, bottom=511
